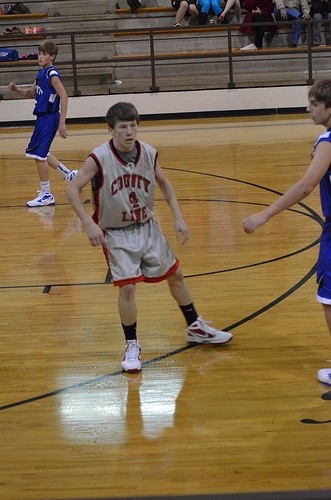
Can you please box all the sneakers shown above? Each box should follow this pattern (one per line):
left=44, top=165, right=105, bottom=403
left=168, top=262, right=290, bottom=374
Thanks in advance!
left=185, top=315, right=233, bottom=343
left=317, top=368, right=331, bottom=386
left=121, top=340, right=141, bottom=372
left=64, top=170, right=78, bottom=183
left=27, top=190, right=55, bottom=207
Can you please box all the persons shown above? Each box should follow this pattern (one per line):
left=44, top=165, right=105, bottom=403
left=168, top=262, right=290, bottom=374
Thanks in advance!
left=171, top=0, right=331, bottom=52
left=66, top=101, right=233, bottom=372
left=244, top=79, right=331, bottom=386
left=7, top=42, right=78, bottom=208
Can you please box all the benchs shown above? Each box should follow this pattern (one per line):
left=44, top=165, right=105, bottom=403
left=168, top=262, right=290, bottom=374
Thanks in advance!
left=0, top=0, right=331, bottom=88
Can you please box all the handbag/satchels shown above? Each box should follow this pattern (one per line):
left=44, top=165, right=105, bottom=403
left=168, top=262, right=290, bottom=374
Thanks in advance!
left=22, top=26, right=46, bottom=34
left=19, top=52, right=38, bottom=59
left=0, top=48, right=19, bottom=61
left=0, top=2, right=32, bottom=14
left=3, top=27, right=22, bottom=35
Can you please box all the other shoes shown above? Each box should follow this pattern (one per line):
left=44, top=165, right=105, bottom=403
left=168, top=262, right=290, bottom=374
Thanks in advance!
left=219, top=13, right=228, bottom=24
left=240, top=43, right=257, bottom=50
left=173, top=22, right=181, bottom=27
